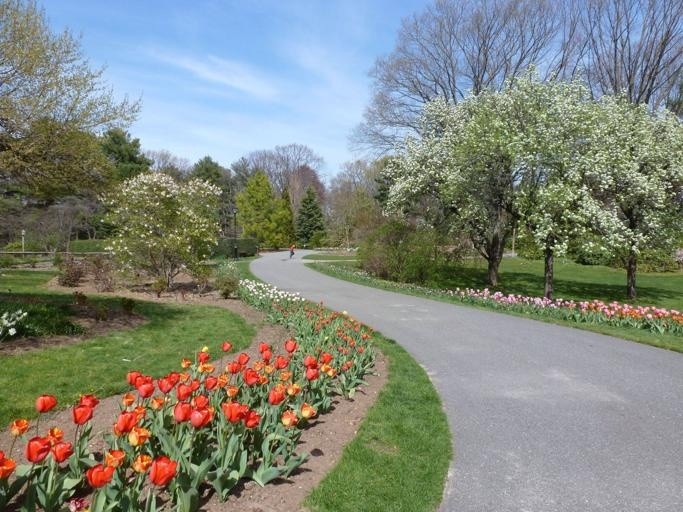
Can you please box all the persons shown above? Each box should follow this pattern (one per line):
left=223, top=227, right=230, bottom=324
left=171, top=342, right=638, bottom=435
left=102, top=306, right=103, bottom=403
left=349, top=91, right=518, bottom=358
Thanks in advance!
left=289, top=244, right=296, bottom=260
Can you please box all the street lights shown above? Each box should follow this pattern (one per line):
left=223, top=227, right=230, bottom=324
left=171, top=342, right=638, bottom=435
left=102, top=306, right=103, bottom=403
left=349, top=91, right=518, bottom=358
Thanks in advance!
left=233, top=207, right=237, bottom=258
